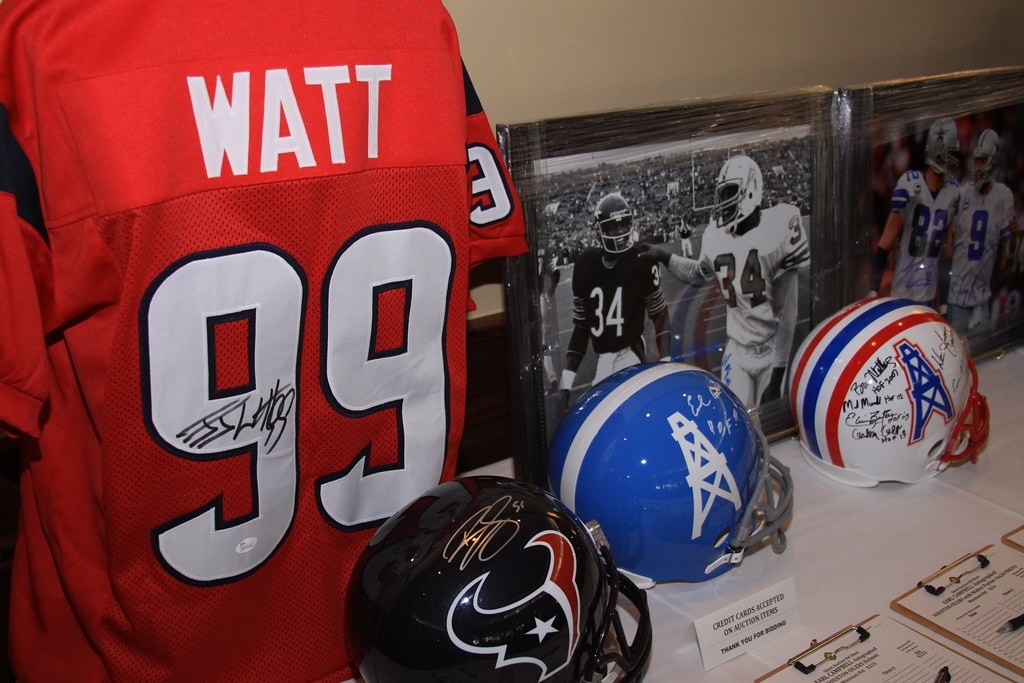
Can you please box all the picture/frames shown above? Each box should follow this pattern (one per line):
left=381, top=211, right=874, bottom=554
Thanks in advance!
left=842, top=67, right=1024, bottom=361
left=497, top=88, right=841, bottom=495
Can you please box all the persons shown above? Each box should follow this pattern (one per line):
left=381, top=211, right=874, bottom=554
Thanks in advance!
left=559, top=192, right=671, bottom=394
left=532, top=136, right=814, bottom=262
left=672, top=220, right=697, bottom=260
left=637, top=153, right=812, bottom=410
left=863, top=117, right=1023, bottom=337
left=537, top=217, right=560, bottom=395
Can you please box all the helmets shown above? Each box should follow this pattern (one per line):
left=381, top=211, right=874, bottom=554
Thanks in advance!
left=925, top=117, right=960, bottom=176
left=544, top=361, right=793, bottom=590
left=973, top=129, right=1001, bottom=182
left=594, top=194, right=634, bottom=253
left=714, top=155, right=762, bottom=234
left=788, top=297, right=990, bottom=488
left=344, top=476, right=653, bottom=683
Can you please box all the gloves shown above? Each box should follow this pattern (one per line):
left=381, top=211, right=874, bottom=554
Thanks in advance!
left=556, top=389, right=569, bottom=413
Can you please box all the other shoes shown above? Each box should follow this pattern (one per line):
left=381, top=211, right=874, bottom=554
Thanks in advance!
left=547, top=374, right=558, bottom=395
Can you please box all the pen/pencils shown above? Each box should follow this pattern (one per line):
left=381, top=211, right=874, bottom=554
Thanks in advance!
left=997, top=613, right=1024, bottom=633
left=934, top=666, right=952, bottom=683
